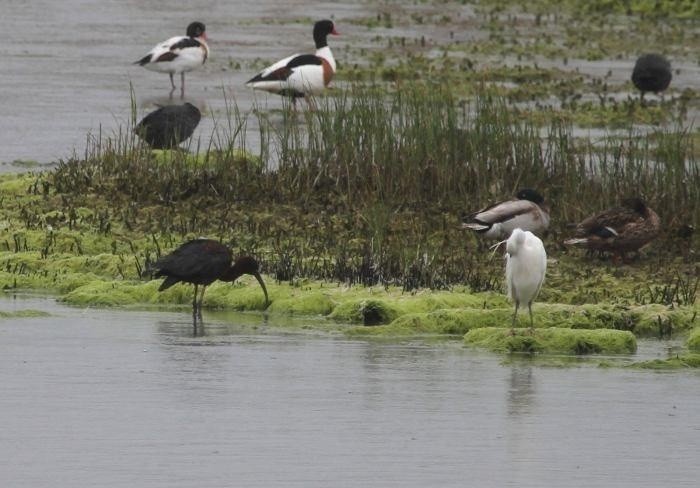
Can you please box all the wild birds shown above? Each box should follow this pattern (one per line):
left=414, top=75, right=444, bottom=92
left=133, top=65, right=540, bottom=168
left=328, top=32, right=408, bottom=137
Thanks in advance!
left=631, top=52, right=673, bottom=100
left=503, top=228, right=548, bottom=337
left=133, top=101, right=201, bottom=149
left=562, top=196, right=663, bottom=265
left=459, top=187, right=552, bottom=245
left=131, top=20, right=212, bottom=90
left=243, top=18, right=341, bottom=111
left=152, top=239, right=269, bottom=324
